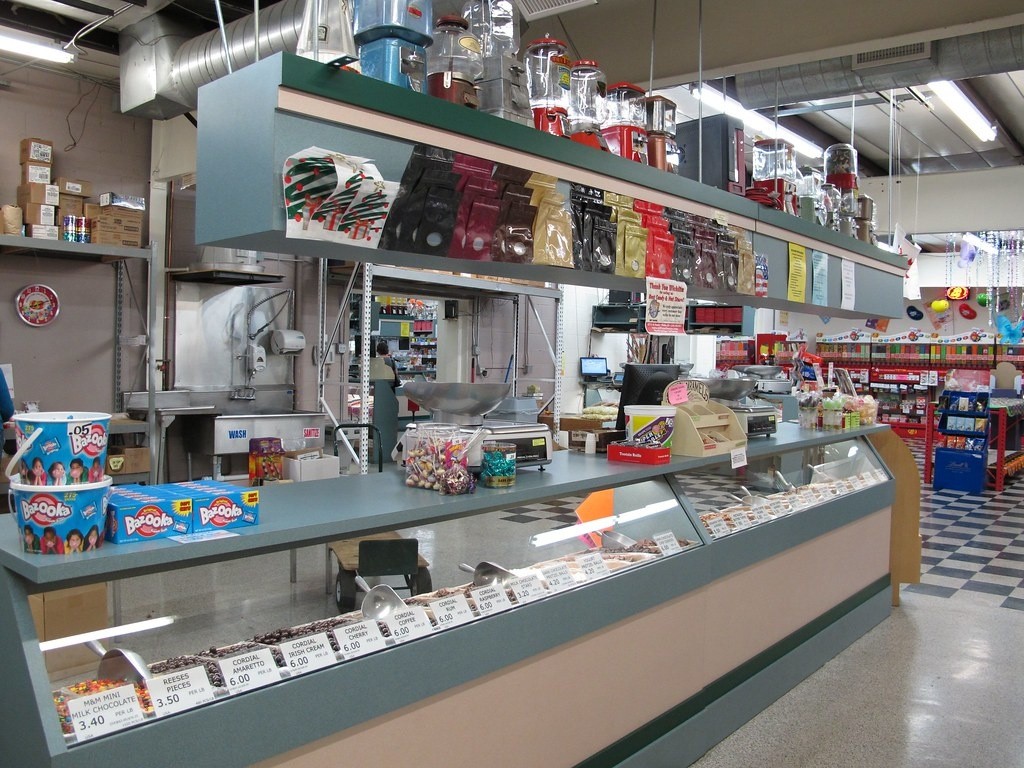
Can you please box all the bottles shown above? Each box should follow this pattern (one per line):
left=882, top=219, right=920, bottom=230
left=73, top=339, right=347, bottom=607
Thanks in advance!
left=63, top=214, right=91, bottom=243
left=523, top=39, right=677, bottom=162
left=405, top=422, right=517, bottom=489
left=752, top=139, right=794, bottom=182
left=796, top=144, right=860, bottom=216
left=425, top=0, right=520, bottom=79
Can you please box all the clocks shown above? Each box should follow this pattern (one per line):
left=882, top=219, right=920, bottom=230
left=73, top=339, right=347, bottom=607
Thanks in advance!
left=15, top=283, right=60, bottom=328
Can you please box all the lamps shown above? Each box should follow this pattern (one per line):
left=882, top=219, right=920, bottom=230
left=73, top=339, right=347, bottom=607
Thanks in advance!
left=927, top=79, right=998, bottom=142
left=527, top=498, right=680, bottom=548
left=688, top=80, right=824, bottom=160
left=961, top=232, right=998, bottom=255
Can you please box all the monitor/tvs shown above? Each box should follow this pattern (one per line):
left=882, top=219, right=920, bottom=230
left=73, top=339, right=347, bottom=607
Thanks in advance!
left=616, top=364, right=680, bottom=431
left=580, top=357, right=607, bottom=382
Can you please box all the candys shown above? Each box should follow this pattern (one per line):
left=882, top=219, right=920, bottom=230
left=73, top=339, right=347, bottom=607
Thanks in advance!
left=54, top=538, right=690, bottom=735
left=407, top=429, right=516, bottom=496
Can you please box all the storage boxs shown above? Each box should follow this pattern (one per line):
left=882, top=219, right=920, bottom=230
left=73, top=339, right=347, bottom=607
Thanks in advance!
left=477, top=54, right=536, bottom=129
left=0, top=454, right=18, bottom=483
left=568, top=427, right=626, bottom=454
left=853, top=193, right=874, bottom=244
left=106, top=479, right=259, bottom=545
left=104, top=444, right=151, bottom=475
left=607, top=444, right=670, bottom=465
left=17, top=138, right=143, bottom=249
left=696, top=308, right=743, bottom=323
left=281, top=447, right=340, bottom=483
left=485, top=396, right=539, bottom=424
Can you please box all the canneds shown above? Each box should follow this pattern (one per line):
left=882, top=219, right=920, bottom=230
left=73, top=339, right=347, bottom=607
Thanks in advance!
left=62, top=215, right=92, bottom=243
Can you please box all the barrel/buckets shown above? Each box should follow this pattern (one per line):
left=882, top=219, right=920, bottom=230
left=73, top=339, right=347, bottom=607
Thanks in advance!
left=5, top=411, right=113, bottom=486
left=8, top=474, right=113, bottom=554
left=624, top=405, right=676, bottom=447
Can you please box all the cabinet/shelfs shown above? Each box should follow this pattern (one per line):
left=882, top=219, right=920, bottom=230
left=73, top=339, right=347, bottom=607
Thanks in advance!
left=0, top=231, right=158, bottom=511
left=591, top=305, right=755, bottom=337
left=776, top=362, right=872, bottom=395
left=869, top=364, right=992, bottom=440
left=410, top=341, right=437, bottom=366
left=933, top=389, right=990, bottom=494
left=924, top=397, right=1024, bottom=491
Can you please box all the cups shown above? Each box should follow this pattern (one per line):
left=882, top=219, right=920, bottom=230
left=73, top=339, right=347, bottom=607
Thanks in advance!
left=585, top=434, right=596, bottom=454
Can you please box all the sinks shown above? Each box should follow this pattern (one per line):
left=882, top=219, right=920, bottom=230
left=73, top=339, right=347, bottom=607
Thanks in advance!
left=186, top=411, right=328, bottom=457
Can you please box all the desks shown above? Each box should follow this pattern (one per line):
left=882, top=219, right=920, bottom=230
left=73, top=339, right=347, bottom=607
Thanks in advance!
left=537, top=412, right=615, bottom=431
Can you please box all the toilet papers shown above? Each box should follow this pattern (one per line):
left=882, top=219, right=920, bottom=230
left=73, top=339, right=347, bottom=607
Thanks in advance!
left=584, top=434, right=596, bottom=454
